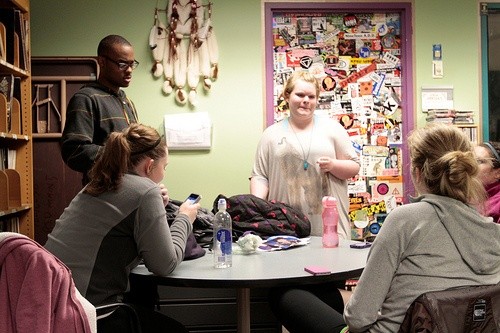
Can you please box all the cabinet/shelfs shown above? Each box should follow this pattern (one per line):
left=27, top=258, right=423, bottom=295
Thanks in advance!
left=0, top=1, right=35, bottom=240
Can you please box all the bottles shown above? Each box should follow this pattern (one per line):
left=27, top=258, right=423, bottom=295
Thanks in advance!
left=213, top=198, right=232, bottom=269
left=322, top=196, right=339, bottom=248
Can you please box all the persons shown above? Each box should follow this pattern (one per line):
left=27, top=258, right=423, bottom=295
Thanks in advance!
left=58, top=35, right=139, bottom=190
left=475, top=141, right=500, bottom=224
left=280, top=124, right=500, bottom=333
left=274, top=237, right=301, bottom=247
left=249, top=70, right=361, bottom=333
left=43, top=123, right=200, bottom=333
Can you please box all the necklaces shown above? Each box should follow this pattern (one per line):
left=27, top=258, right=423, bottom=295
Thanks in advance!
left=289, top=117, right=315, bottom=171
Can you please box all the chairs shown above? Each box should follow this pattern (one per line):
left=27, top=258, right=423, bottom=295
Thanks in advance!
left=0, top=231, right=138, bottom=333
left=398, top=283, right=499, bottom=333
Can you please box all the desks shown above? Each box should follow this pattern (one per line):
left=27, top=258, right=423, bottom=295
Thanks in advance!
left=131, top=236, right=371, bottom=333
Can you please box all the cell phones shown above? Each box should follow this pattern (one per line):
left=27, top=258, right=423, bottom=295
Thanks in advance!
left=173, top=193, right=202, bottom=216
left=304, top=265, right=331, bottom=276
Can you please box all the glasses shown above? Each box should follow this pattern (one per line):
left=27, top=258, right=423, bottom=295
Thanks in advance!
left=102, top=55, right=139, bottom=71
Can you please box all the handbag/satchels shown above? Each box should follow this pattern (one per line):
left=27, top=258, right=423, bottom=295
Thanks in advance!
left=212, top=194, right=311, bottom=240
